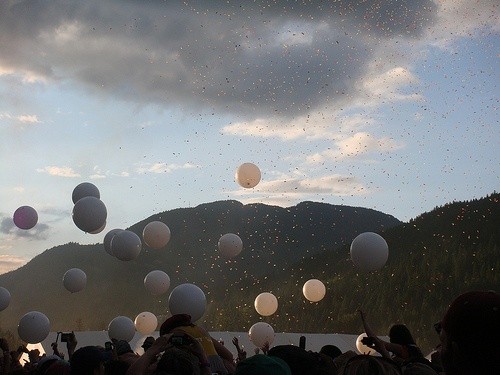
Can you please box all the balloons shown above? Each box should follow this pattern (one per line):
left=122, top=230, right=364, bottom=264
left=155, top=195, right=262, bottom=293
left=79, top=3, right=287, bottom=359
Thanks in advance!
left=134, top=312, right=156, bottom=334
left=217, top=234, right=243, bottom=257
left=72, top=182, right=100, bottom=204
left=143, top=220, right=171, bottom=250
left=0, top=287, right=10, bottom=312
left=103, top=229, right=141, bottom=261
left=238, top=163, right=261, bottom=188
left=18, top=312, right=49, bottom=344
left=63, top=269, right=87, bottom=293
left=168, top=284, right=207, bottom=321
left=109, top=316, right=135, bottom=343
left=249, top=321, right=274, bottom=347
left=350, top=232, right=389, bottom=271
left=356, top=333, right=376, bottom=355
left=71, top=196, right=107, bottom=234
left=13, top=205, right=38, bottom=231
left=254, top=293, right=278, bottom=316
left=303, top=280, right=325, bottom=302
left=144, top=270, right=170, bottom=298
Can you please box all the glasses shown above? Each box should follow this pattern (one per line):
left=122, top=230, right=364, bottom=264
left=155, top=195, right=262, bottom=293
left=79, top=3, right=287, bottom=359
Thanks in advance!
left=433, top=322, right=446, bottom=335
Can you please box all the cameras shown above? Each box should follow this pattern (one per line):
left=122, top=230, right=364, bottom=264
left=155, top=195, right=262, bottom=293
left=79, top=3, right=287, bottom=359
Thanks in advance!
left=362, top=337, right=373, bottom=346
left=61, top=333, right=72, bottom=342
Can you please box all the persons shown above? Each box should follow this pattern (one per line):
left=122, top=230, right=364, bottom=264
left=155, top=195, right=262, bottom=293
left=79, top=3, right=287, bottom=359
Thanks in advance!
left=433, top=291, right=500, bottom=375
left=0, top=306, right=435, bottom=375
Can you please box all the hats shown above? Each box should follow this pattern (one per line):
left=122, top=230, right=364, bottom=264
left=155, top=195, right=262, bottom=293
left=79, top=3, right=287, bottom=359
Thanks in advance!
left=38, top=355, right=61, bottom=367
left=442, top=291, right=500, bottom=345
left=160, top=314, right=192, bottom=336
left=70, top=346, right=112, bottom=372
left=141, top=337, right=155, bottom=348
left=235, top=354, right=291, bottom=375
